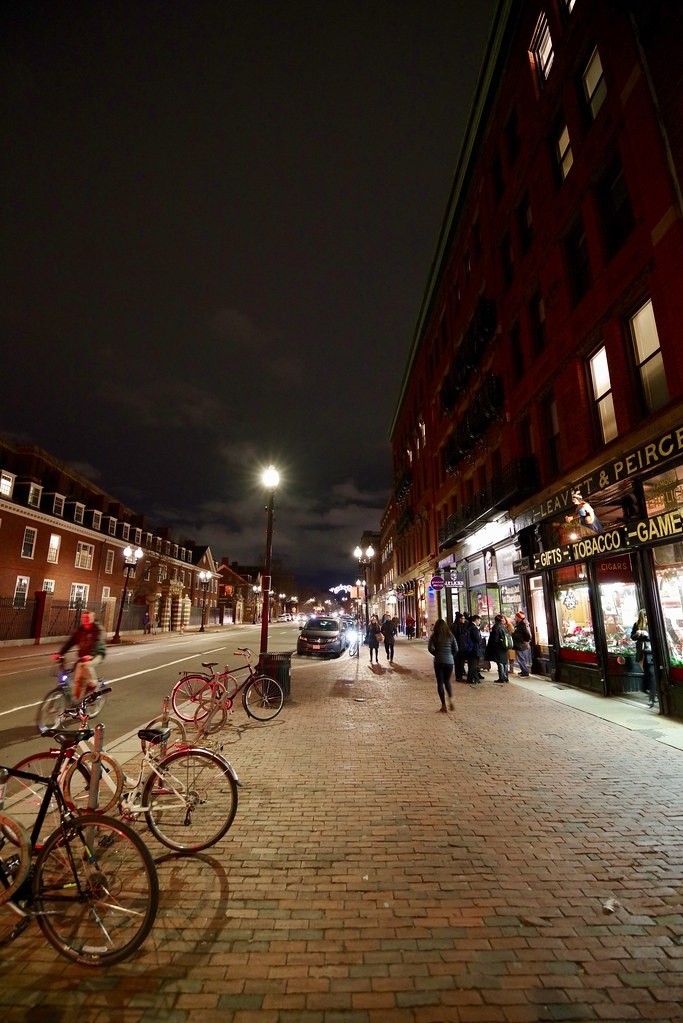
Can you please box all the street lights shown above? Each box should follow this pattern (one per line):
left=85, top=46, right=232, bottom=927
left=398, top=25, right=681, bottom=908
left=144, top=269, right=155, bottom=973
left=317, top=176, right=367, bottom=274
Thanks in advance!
left=354, top=544, right=375, bottom=645
left=199, top=570, right=212, bottom=631
left=252, top=585, right=285, bottom=624
left=111, top=546, right=144, bottom=644
left=251, top=464, right=281, bottom=675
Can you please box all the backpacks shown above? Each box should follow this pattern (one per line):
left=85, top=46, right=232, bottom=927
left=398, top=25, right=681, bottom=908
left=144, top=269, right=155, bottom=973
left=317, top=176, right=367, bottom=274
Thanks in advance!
left=497, top=626, right=514, bottom=648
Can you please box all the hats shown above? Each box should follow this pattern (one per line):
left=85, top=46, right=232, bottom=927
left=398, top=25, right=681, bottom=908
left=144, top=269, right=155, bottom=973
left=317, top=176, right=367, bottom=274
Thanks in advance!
left=463, top=611, right=471, bottom=618
left=455, top=611, right=464, bottom=621
left=516, top=611, right=525, bottom=620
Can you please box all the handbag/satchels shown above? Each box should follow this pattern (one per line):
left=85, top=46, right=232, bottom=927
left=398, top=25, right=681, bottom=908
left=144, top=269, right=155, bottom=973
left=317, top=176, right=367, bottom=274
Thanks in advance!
left=642, top=641, right=652, bottom=652
left=375, top=632, right=385, bottom=643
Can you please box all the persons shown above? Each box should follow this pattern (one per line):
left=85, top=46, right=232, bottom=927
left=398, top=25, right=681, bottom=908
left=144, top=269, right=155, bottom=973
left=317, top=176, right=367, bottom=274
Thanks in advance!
left=565, top=490, right=603, bottom=536
left=406, top=614, right=415, bottom=640
left=142, top=613, right=149, bottom=633
left=381, top=611, right=389, bottom=623
left=51, top=611, right=106, bottom=700
left=666, top=618, right=680, bottom=644
left=365, top=617, right=380, bottom=663
left=381, top=615, right=397, bottom=662
left=484, top=615, right=509, bottom=683
left=392, top=614, right=400, bottom=636
left=428, top=618, right=459, bottom=712
left=502, top=616, right=514, bottom=673
left=512, top=611, right=532, bottom=677
left=630, top=608, right=656, bottom=707
left=450, top=612, right=485, bottom=684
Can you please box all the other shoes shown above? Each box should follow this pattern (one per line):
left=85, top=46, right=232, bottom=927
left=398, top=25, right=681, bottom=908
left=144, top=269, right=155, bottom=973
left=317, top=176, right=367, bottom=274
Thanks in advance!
left=507, top=670, right=513, bottom=673
left=521, top=673, right=529, bottom=677
left=87, top=683, right=98, bottom=693
left=457, top=678, right=466, bottom=683
left=462, top=671, right=469, bottom=676
left=518, top=672, right=523, bottom=675
left=440, top=704, right=447, bottom=711
left=478, top=673, right=485, bottom=679
left=652, top=692, right=659, bottom=702
left=494, top=677, right=510, bottom=683
left=642, top=689, right=650, bottom=694
left=449, top=703, right=454, bottom=711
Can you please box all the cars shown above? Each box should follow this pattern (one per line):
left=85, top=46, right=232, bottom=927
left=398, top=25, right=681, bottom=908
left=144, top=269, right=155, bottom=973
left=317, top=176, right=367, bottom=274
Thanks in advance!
left=278, top=611, right=355, bottom=659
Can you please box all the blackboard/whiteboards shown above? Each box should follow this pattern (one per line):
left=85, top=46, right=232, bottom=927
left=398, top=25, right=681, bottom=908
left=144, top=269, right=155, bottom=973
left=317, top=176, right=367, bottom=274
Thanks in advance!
left=478, top=638, right=491, bottom=669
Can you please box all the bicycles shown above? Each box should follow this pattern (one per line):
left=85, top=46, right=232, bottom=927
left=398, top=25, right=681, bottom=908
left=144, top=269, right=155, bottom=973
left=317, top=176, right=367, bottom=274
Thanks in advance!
left=171, top=648, right=285, bottom=723
left=349, top=632, right=360, bottom=656
left=36, top=658, right=108, bottom=733
left=0, top=688, right=243, bottom=968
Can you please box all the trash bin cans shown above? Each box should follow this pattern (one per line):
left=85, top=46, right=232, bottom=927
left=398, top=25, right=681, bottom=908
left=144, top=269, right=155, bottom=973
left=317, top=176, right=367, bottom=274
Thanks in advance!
left=262, top=652, right=293, bottom=697
left=253, top=619, right=256, bottom=624
